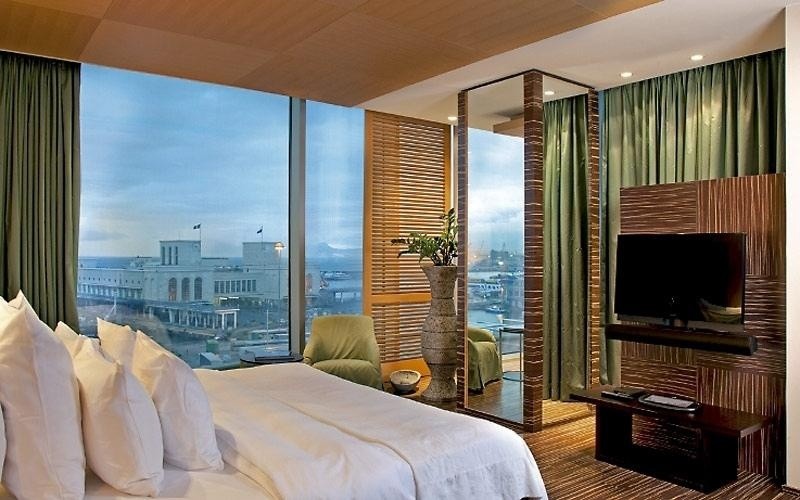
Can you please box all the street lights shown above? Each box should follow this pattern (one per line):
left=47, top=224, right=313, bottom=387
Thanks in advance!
left=274, top=242, right=284, bottom=329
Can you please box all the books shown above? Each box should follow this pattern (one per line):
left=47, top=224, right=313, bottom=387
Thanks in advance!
left=253, top=350, right=295, bottom=362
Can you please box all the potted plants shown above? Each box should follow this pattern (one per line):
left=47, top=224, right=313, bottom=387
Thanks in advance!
left=390, top=208, right=459, bottom=403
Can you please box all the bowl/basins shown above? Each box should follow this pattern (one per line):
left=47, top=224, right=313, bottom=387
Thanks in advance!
left=389, top=369, right=421, bottom=391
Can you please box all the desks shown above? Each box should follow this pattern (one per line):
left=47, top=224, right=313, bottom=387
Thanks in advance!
left=568, top=384, right=773, bottom=494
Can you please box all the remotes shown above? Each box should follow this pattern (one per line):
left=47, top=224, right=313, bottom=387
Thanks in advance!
left=600, top=390, right=635, bottom=402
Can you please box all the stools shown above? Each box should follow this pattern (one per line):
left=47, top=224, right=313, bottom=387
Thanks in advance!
left=497, top=326, right=524, bottom=383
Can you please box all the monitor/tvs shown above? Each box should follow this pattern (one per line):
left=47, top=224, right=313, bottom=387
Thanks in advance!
left=613, top=231, right=748, bottom=333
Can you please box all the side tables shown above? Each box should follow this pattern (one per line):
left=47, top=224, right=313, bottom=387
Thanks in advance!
left=240, top=350, right=303, bottom=365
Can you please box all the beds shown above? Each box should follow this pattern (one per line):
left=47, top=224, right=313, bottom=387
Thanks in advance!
left=1, top=288, right=549, bottom=500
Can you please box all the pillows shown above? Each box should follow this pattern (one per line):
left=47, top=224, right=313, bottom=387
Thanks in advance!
left=0, top=291, right=86, bottom=497
left=56, top=321, right=165, bottom=498
left=132, top=330, right=224, bottom=471
left=93, top=318, right=134, bottom=368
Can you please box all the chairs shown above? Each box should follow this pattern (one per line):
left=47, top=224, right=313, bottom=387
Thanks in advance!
left=466, top=326, right=504, bottom=397
left=303, top=314, right=383, bottom=390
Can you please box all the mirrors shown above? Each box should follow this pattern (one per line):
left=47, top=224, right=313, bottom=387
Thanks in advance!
left=542, top=72, right=590, bottom=424
left=467, top=72, right=524, bottom=425
